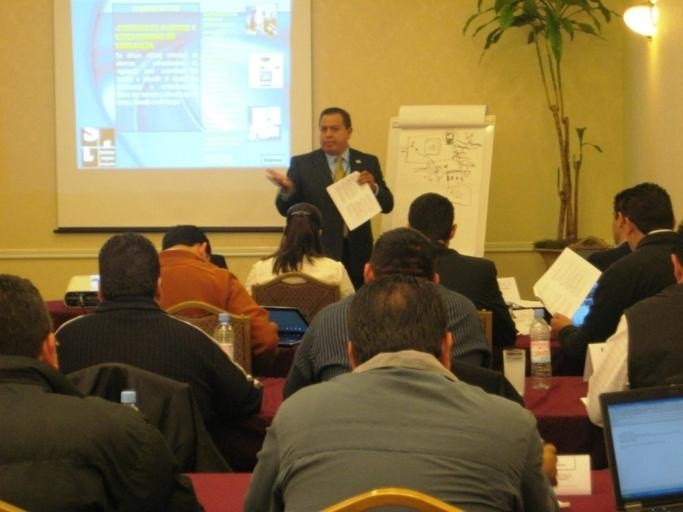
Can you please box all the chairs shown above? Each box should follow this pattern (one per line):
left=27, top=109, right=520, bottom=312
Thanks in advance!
left=252, top=270, right=342, bottom=326
left=478, top=310, right=492, bottom=352
left=65, top=362, right=234, bottom=473
left=449, top=358, right=526, bottom=406
left=164, top=300, right=252, bottom=377
left=321, top=486, right=466, bottom=512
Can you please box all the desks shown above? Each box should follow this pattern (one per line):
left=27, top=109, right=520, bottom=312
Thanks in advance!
left=516, top=331, right=562, bottom=376
left=183, top=468, right=618, bottom=512
left=43, top=298, right=95, bottom=330
left=249, top=375, right=607, bottom=469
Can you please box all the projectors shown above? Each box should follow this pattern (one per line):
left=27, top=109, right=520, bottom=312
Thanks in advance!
left=64, top=275, right=101, bottom=307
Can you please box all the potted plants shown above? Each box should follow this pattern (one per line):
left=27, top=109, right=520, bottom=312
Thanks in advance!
left=461, top=0, right=622, bottom=271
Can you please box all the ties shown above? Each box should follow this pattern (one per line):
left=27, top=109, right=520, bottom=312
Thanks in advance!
left=334, top=157, right=349, bottom=237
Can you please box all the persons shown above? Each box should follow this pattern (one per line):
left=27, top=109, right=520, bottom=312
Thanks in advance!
left=241, top=202, right=357, bottom=300
left=586, top=219, right=683, bottom=431
left=263, top=108, right=394, bottom=282
left=408, top=193, right=517, bottom=350
left=543, top=188, right=634, bottom=326
left=155, top=224, right=280, bottom=373
left=242, top=276, right=563, bottom=512
left=0, top=273, right=205, bottom=512
left=53, top=233, right=263, bottom=473
left=281, top=227, right=493, bottom=399
left=549, top=182, right=676, bottom=376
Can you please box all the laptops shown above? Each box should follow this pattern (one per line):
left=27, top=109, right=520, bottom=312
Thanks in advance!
left=599, top=385, right=683, bottom=512
left=544, top=278, right=599, bottom=328
left=260, top=306, right=310, bottom=347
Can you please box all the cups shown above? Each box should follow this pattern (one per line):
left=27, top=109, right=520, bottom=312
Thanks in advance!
left=502, top=348, right=526, bottom=401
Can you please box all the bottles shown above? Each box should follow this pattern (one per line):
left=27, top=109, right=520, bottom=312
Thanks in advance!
left=528, top=308, right=553, bottom=391
left=119, top=390, right=140, bottom=413
left=213, top=311, right=234, bottom=362
